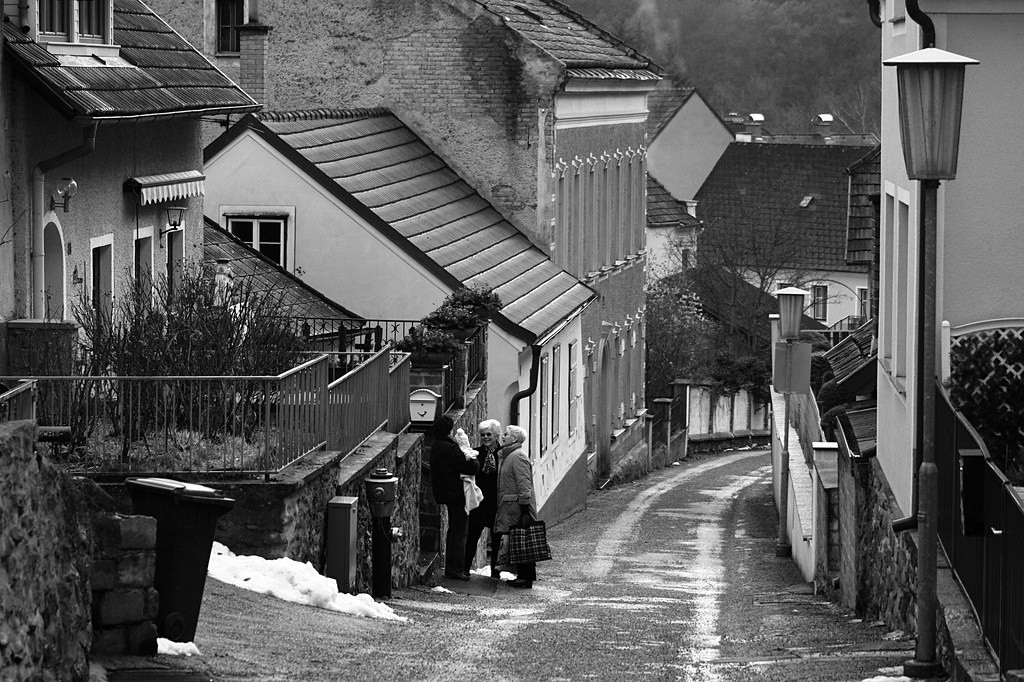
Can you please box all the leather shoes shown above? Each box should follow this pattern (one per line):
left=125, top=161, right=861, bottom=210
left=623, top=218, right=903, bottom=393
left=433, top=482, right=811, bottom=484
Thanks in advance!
left=506, top=568, right=536, bottom=589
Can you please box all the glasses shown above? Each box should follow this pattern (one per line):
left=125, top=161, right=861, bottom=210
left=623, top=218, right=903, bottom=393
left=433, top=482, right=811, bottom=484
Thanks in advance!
left=503, top=432, right=511, bottom=436
left=481, top=432, right=493, bottom=437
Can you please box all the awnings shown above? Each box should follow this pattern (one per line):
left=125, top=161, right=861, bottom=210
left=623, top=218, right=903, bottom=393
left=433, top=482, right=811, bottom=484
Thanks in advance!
left=130, top=170, right=205, bottom=205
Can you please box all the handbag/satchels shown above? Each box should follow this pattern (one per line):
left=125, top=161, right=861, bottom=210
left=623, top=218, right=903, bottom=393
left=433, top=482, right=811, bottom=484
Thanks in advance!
left=509, top=515, right=552, bottom=565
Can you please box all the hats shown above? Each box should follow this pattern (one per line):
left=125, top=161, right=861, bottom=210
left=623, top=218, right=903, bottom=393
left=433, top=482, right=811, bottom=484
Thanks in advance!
left=433, top=415, right=454, bottom=436
left=454, top=429, right=470, bottom=446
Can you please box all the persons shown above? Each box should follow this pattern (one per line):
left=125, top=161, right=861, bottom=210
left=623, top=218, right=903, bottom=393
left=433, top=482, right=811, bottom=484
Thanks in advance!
left=454, top=428, right=484, bottom=515
left=465, top=419, right=501, bottom=578
left=430, top=416, right=478, bottom=580
left=494, top=425, right=538, bottom=589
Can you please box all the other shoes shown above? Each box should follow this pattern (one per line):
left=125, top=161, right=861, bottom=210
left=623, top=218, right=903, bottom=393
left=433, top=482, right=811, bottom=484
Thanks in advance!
left=445, top=571, right=472, bottom=582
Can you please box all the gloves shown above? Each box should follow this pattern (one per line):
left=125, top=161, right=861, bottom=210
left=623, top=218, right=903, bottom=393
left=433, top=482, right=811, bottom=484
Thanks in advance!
left=521, top=503, right=530, bottom=515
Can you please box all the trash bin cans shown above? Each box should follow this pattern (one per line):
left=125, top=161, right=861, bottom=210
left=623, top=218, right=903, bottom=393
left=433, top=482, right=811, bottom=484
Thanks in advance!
left=122, top=476, right=237, bottom=642
